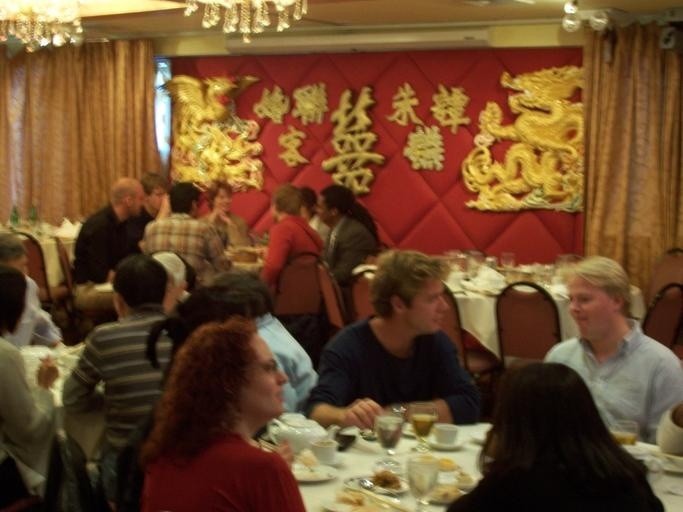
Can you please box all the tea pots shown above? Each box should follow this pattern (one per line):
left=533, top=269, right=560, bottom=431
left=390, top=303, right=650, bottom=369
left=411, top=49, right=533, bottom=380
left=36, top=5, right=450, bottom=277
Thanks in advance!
left=266, top=413, right=337, bottom=451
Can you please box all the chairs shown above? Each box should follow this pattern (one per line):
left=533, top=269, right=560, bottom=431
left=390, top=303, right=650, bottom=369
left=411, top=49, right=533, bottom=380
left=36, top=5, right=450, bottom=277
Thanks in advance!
left=1, top=222, right=273, bottom=432
left=42, top=430, right=108, bottom=510
left=1, top=446, right=39, bottom=510
left=347, top=266, right=379, bottom=323
left=314, top=261, right=350, bottom=333
left=495, top=281, right=561, bottom=371
left=642, top=282, right=680, bottom=350
left=441, top=283, right=494, bottom=376
left=273, top=252, right=318, bottom=322
left=644, top=246, right=680, bottom=310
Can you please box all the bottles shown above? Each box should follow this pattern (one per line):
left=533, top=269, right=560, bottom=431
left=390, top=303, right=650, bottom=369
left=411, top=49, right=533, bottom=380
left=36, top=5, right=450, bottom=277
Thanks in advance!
left=10, top=206, right=19, bottom=224
left=262, top=230, right=268, bottom=245
left=30, top=205, right=36, bottom=224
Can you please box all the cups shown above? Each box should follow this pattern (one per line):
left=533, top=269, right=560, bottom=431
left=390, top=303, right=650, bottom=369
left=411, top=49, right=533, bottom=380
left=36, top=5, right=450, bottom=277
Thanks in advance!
left=38, top=215, right=80, bottom=239
left=609, top=421, right=638, bottom=444
left=308, top=400, right=494, bottom=511
left=59, top=354, right=81, bottom=369
left=436, top=248, right=559, bottom=295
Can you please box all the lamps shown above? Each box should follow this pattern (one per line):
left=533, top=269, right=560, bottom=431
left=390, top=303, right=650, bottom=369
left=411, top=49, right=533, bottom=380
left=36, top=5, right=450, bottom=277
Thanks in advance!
left=561, top=0, right=610, bottom=34
left=178, top=0, right=312, bottom=48
left=0, top=0, right=85, bottom=52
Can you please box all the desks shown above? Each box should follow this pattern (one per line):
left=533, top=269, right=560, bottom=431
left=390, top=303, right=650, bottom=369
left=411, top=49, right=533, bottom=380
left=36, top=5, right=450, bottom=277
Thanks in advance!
left=444, top=284, right=642, bottom=363
left=297, top=420, right=681, bottom=510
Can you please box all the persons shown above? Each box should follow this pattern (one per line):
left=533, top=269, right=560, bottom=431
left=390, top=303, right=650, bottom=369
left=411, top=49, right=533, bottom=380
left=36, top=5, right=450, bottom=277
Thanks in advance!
left=0, top=173, right=381, bottom=510
left=446, top=361, right=664, bottom=512
left=541, top=256, right=682, bottom=444
left=656, top=404, right=683, bottom=457
left=302, top=248, right=482, bottom=427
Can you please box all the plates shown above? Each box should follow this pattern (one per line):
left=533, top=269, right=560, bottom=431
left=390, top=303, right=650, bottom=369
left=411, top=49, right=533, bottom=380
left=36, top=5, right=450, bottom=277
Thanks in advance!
left=644, top=451, right=683, bottom=493
left=289, top=462, right=340, bottom=483
